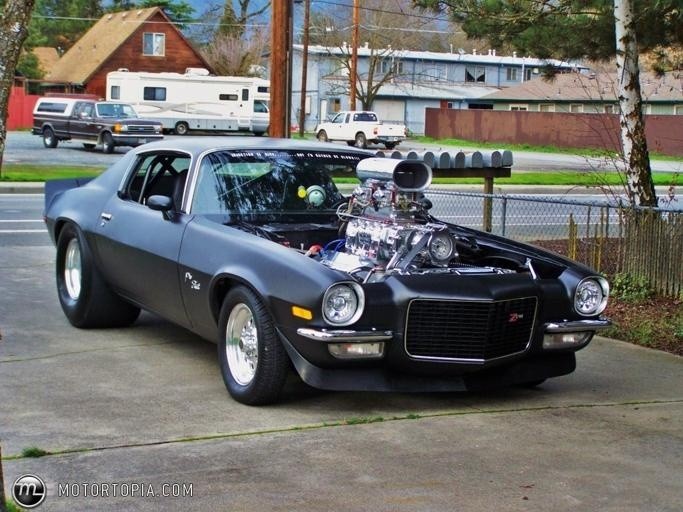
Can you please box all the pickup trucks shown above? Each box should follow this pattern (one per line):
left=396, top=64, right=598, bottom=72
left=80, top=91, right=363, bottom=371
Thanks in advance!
left=315, top=111, right=406, bottom=149
left=32, top=97, right=164, bottom=153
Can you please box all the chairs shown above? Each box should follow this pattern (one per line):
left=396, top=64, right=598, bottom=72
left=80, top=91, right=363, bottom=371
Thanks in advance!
left=173, top=167, right=189, bottom=215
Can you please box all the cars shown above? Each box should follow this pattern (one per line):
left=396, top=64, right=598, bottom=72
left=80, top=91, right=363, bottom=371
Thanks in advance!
left=44, top=136, right=611, bottom=407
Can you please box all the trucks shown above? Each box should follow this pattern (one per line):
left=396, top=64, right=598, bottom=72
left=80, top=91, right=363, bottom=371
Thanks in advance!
left=105, top=67, right=271, bottom=137
